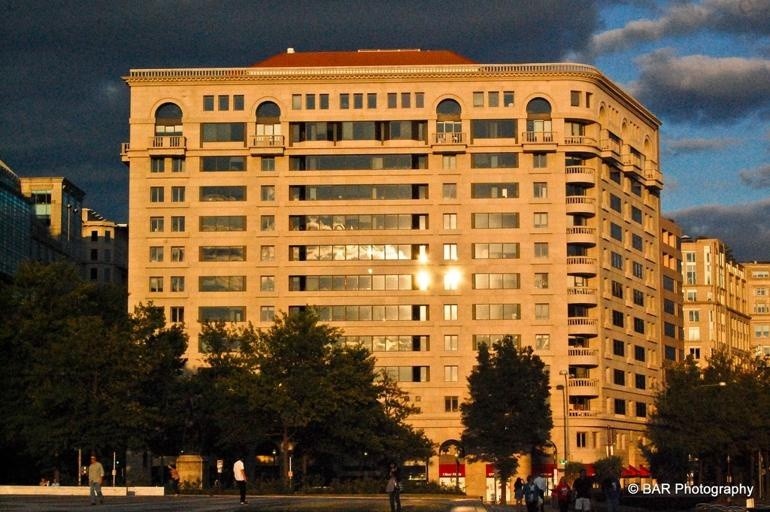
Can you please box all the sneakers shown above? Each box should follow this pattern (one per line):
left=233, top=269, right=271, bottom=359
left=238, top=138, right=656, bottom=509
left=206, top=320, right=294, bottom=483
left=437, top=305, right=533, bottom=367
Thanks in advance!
left=241, top=500, right=248, bottom=505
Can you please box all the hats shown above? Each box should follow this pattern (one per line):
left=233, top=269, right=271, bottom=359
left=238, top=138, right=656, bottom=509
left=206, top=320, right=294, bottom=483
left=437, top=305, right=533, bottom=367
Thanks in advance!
left=579, top=469, right=585, bottom=472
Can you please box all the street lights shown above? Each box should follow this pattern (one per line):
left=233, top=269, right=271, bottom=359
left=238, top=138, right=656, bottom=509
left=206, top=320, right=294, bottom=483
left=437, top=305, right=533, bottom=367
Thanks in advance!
left=559, top=370, right=569, bottom=475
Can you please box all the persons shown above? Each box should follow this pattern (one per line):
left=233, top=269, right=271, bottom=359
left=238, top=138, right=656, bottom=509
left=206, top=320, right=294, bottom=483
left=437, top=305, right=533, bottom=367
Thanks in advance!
left=51, top=478, right=60, bottom=486
left=233, top=456, right=249, bottom=505
left=512, top=468, right=621, bottom=512
left=88, top=456, right=105, bottom=505
left=168, top=463, right=180, bottom=497
left=384, top=458, right=401, bottom=512
left=39, top=478, right=49, bottom=486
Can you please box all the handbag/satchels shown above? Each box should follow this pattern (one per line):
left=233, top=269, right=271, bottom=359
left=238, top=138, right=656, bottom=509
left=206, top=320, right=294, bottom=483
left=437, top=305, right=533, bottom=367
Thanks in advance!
left=538, top=497, right=543, bottom=507
